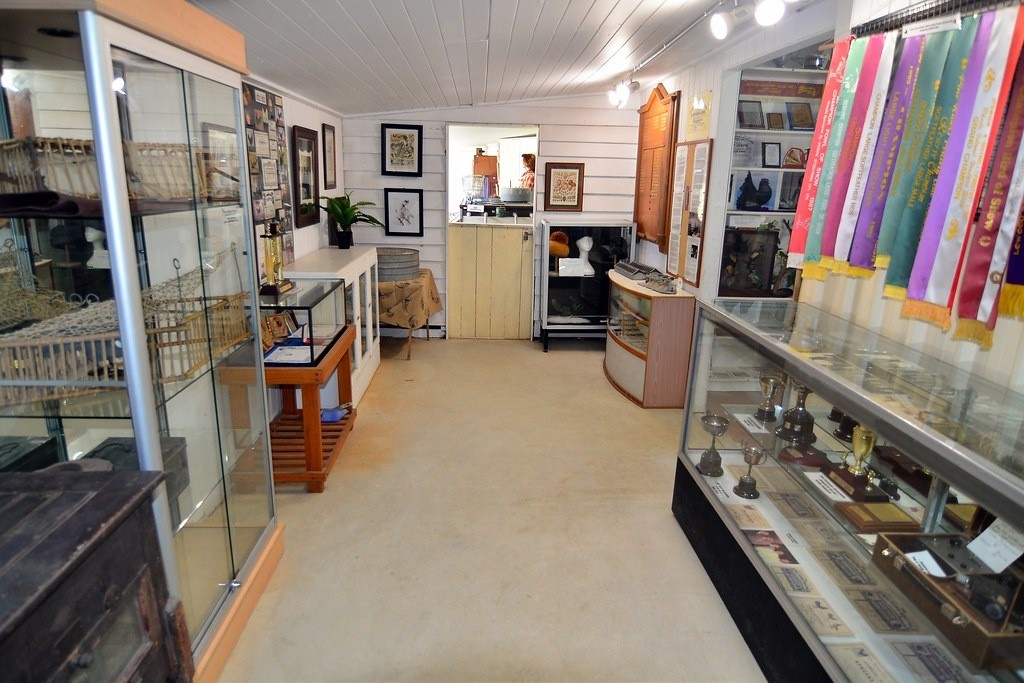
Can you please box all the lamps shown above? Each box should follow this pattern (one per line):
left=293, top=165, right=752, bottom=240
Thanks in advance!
left=609, top=75, right=640, bottom=106
left=711, top=0, right=755, bottom=40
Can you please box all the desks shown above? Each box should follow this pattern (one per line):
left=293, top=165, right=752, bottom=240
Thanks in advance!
left=378, top=269, right=443, bottom=360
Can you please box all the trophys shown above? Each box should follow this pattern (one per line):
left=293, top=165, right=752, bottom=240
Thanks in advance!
left=847, top=425, right=877, bottom=476
left=733, top=440, right=767, bottom=499
left=754, top=376, right=785, bottom=421
left=259, top=222, right=293, bottom=295
left=775, top=377, right=817, bottom=444
left=695, top=409, right=729, bottom=477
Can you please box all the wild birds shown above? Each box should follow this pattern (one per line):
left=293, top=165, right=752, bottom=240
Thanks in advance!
left=735, top=171, right=772, bottom=207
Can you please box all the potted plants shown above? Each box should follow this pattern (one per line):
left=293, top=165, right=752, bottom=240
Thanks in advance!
left=317, top=191, right=385, bottom=249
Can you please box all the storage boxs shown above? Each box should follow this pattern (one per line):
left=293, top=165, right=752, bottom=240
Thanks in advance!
left=873, top=503, right=1023, bottom=670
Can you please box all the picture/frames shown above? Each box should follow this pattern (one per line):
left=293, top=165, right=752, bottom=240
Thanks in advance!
left=381, top=123, right=423, bottom=177
left=384, top=188, right=423, bottom=236
left=293, top=123, right=337, bottom=228
left=544, top=163, right=585, bottom=212
left=738, top=100, right=765, bottom=129
left=766, top=113, right=784, bottom=130
left=786, top=102, right=815, bottom=131
left=202, top=122, right=240, bottom=201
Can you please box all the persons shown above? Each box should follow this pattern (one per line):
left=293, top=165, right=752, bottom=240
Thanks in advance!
left=85, top=219, right=105, bottom=250
left=573, top=230, right=595, bottom=275
left=743, top=530, right=790, bottom=564
left=520, top=153, right=535, bottom=188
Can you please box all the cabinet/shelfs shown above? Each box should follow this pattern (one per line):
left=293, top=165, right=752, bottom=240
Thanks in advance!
left=726, top=128, right=814, bottom=216
left=671, top=298, right=1024, bottom=683
left=283, top=247, right=380, bottom=410
left=218, top=323, right=357, bottom=494
left=540, top=218, right=637, bottom=353
left=604, top=268, right=696, bottom=409
left=0, top=0, right=286, bottom=683
